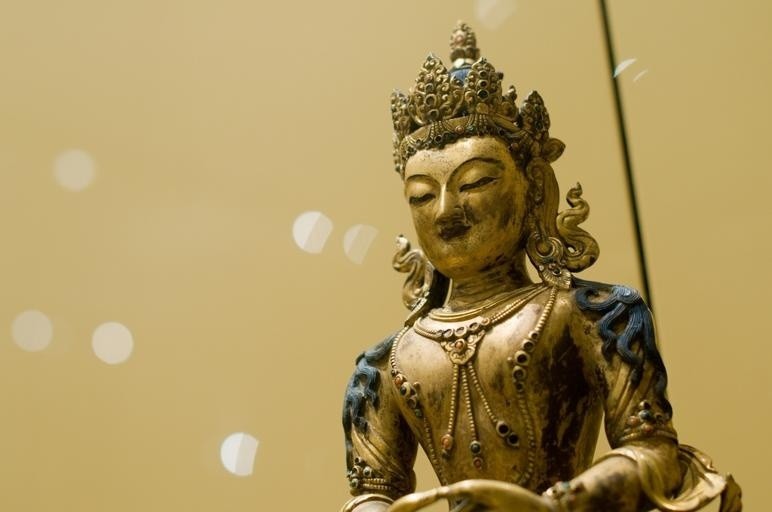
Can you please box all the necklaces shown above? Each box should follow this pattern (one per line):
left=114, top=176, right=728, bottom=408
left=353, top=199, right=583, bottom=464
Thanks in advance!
left=389, top=279, right=559, bottom=488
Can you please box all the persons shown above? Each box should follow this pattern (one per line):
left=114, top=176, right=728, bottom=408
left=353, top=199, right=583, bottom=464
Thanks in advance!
left=341, top=20, right=726, bottom=512
